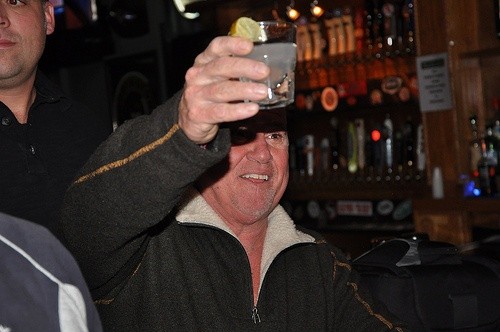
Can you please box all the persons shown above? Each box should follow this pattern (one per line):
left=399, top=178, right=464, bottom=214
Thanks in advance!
left=60, top=37, right=408, bottom=332
left=0, top=0, right=112, bottom=238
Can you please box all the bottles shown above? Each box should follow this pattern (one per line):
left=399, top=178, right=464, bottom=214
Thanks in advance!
left=273, top=0, right=415, bottom=74
left=461, top=95, right=500, bottom=198
left=289, top=113, right=426, bottom=183
left=432, top=164, right=445, bottom=200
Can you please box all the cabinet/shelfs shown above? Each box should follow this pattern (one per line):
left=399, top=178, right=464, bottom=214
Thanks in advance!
left=216, top=0, right=500, bottom=255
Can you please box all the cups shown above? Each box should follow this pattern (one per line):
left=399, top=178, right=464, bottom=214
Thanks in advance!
left=227, top=21, right=297, bottom=110
